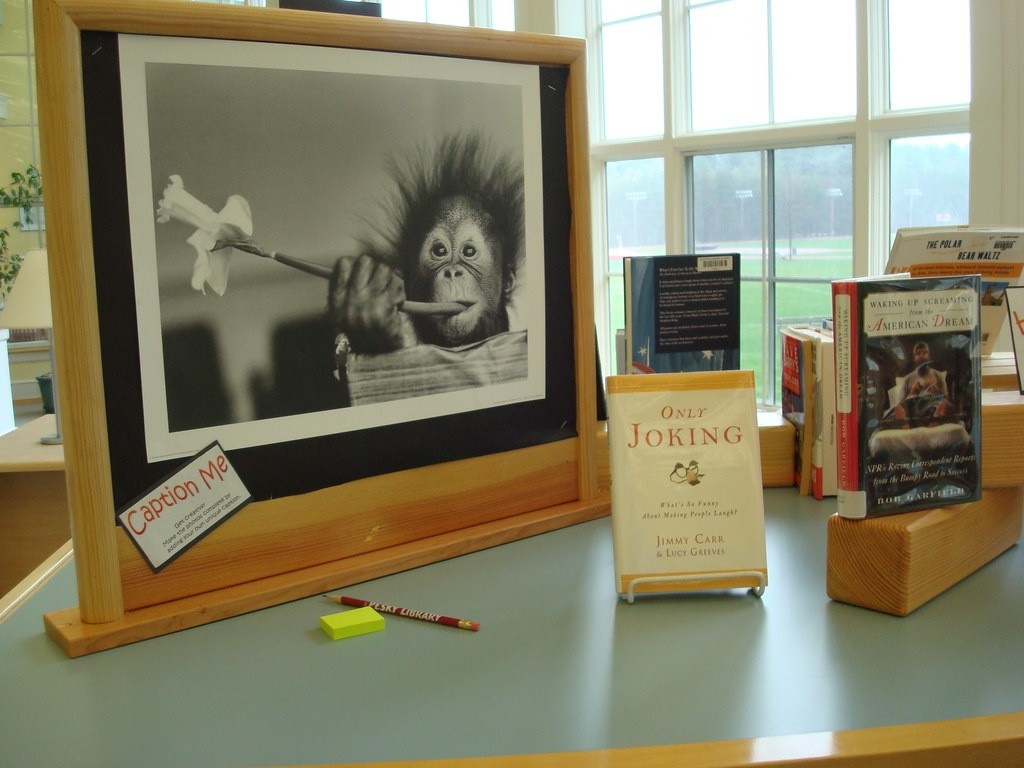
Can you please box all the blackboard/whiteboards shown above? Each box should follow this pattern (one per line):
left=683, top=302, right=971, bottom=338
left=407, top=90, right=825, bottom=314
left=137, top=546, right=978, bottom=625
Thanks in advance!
left=0, top=248, right=51, bottom=329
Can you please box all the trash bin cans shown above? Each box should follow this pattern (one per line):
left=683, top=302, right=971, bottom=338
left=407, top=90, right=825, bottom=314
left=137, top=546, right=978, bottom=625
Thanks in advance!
left=36, top=372, right=54, bottom=414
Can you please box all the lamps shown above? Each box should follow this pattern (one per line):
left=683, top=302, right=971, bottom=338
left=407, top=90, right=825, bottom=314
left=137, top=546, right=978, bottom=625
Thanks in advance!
left=0, top=249, right=63, bottom=445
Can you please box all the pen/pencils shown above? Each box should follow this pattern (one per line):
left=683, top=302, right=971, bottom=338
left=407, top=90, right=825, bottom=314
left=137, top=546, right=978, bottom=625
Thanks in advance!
left=323, top=594, right=480, bottom=631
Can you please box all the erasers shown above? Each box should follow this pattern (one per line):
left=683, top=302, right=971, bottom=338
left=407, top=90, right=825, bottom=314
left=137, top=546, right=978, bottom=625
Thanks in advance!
left=319, top=606, right=385, bottom=639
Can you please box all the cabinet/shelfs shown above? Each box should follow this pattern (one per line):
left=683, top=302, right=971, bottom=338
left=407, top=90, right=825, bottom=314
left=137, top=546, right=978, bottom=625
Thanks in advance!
left=0, top=414, right=74, bottom=597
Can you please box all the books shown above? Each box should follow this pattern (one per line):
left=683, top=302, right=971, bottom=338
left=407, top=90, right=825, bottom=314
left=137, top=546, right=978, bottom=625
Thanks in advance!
left=782, top=225, right=1024, bottom=518
left=604, top=253, right=769, bottom=594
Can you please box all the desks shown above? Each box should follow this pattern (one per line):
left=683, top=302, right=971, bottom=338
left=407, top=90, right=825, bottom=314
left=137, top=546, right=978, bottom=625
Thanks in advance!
left=0, top=486, right=1024, bottom=768
